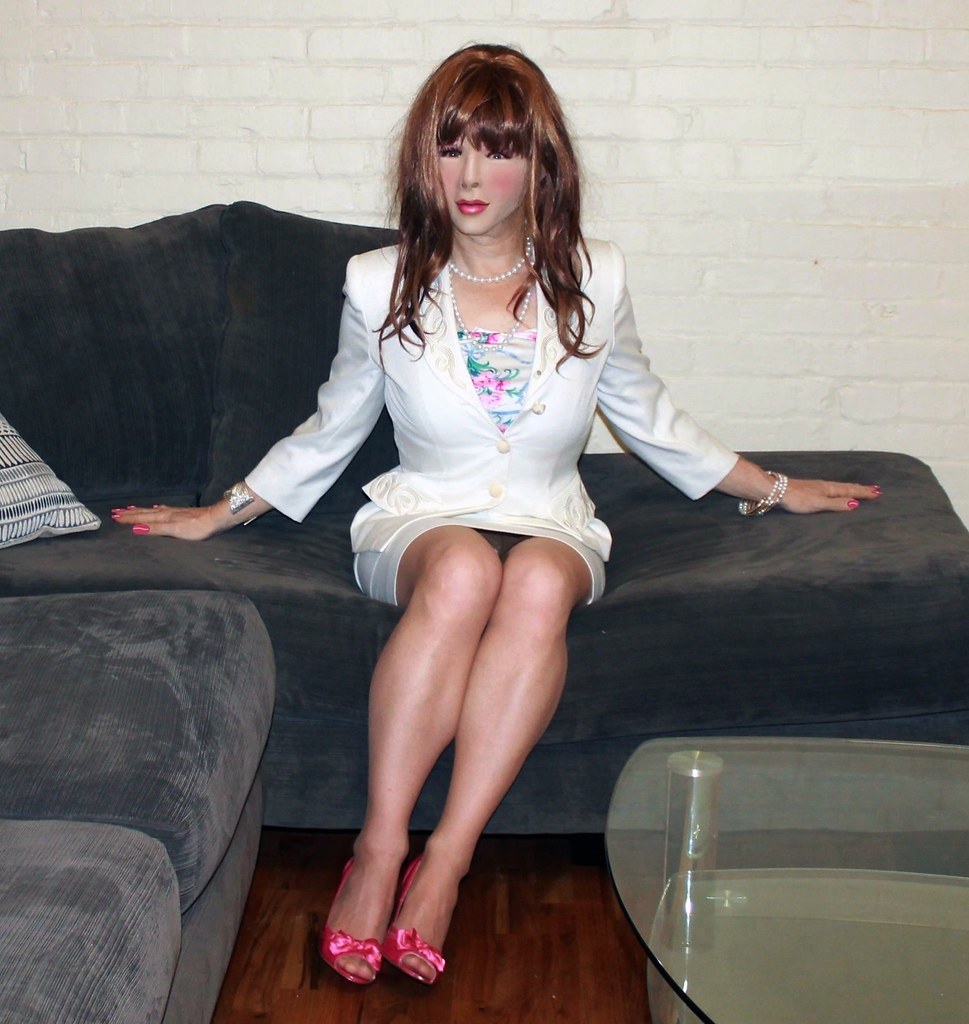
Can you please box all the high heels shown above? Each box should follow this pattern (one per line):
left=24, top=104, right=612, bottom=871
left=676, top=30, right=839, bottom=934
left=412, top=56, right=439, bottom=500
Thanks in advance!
left=319, top=857, right=383, bottom=985
left=381, top=853, right=446, bottom=985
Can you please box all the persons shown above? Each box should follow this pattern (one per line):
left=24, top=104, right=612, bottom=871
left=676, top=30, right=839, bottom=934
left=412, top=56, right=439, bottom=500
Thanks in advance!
left=111, top=44, right=884, bottom=992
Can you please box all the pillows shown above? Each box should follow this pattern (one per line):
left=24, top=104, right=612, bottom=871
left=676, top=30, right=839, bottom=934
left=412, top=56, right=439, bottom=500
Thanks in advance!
left=0, top=412, right=101, bottom=548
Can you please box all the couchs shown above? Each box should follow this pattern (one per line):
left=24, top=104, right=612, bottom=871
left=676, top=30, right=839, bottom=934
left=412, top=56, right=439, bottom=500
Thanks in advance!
left=0, top=203, right=969, bottom=1024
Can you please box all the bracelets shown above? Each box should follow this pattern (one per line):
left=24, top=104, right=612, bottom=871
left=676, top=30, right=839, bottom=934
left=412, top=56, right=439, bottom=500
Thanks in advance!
left=738, top=472, right=788, bottom=516
left=223, top=481, right=257, bottom=525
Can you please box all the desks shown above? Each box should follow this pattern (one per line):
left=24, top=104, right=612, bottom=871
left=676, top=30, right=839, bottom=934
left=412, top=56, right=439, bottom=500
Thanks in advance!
left=607, top=734, right=969, bottom=1024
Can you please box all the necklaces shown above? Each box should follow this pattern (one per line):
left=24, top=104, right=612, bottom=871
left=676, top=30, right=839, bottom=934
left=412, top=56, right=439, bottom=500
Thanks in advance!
left=448, top=227, right=534, bottom=351
